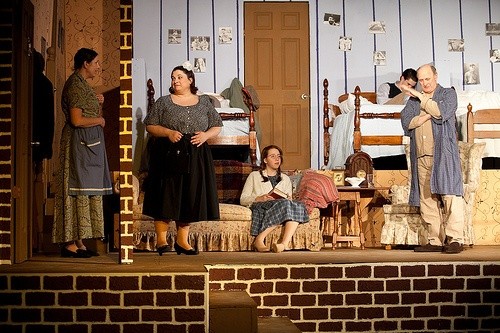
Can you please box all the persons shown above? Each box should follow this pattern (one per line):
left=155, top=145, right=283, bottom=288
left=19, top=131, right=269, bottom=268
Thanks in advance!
left=325, top=15, right=500, bottom=84
left=142, top=60, right=225, bottom=256
left=52, top=48, right=113, bottom=257
left=371, top=68, right=418, bottom=170
left=240, top=145, right=309, bottom=253
left=399, top=64, right=465, bottom=254
left=170, top=29, right=231, bottom=72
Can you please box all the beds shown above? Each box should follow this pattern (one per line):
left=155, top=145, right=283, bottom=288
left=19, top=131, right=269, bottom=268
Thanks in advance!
left=322, top=78, right=474, bottom=170
left=455, top=89, right=500, bottom=171
left=145, top=78, right=257, bottom=167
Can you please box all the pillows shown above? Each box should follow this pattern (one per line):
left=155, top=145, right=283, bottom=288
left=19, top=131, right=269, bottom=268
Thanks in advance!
left=133, top=202, right=156, bottom=221
left=197, top=91, right=233, bottom=108
left=338, top=93, right=374, bottom=114
left=218, top=203, right=252, bottom=221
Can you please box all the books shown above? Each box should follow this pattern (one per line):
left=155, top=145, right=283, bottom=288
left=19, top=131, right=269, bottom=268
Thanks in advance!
left=267, top=188, right=288, bottom=199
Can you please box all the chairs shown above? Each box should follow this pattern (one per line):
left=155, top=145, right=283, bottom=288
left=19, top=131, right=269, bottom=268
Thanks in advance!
left=466, top=102, right=500, bottom=170
left=384, top=142, right=487, bottom=250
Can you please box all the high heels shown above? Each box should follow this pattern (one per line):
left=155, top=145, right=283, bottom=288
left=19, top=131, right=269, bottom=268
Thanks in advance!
left=156, top=244, right=171, bottom=256
left=174, top=242, right=198, bottom=255
left=272, top=242, right=284, bottom=252
left=252, top=241, right=270, bottom=253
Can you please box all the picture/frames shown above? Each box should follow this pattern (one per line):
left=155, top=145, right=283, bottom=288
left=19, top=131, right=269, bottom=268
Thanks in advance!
left=58, top=20, right=66, bottom=53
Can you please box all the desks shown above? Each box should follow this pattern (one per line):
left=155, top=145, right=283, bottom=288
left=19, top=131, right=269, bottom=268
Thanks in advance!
left=325, top=187, right=390, bottom=251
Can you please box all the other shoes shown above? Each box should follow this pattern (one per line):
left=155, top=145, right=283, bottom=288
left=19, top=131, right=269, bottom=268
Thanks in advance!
left=61, top=246, right=100, bottom=258
left=414, top=242, right=445, bottom=252
left=445, top=242, right=463, bottom=252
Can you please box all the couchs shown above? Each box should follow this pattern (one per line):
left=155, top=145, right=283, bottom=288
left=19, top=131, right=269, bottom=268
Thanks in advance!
left=133, top=160, right=323, bottom=252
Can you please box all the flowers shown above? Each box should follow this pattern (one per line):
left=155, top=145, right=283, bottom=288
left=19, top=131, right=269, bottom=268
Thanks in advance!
left=183, top=60, right=194, bottom=72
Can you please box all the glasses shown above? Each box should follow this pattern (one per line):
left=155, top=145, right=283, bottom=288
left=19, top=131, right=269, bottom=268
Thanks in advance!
left=267, top=153, right=281, bottom=159
